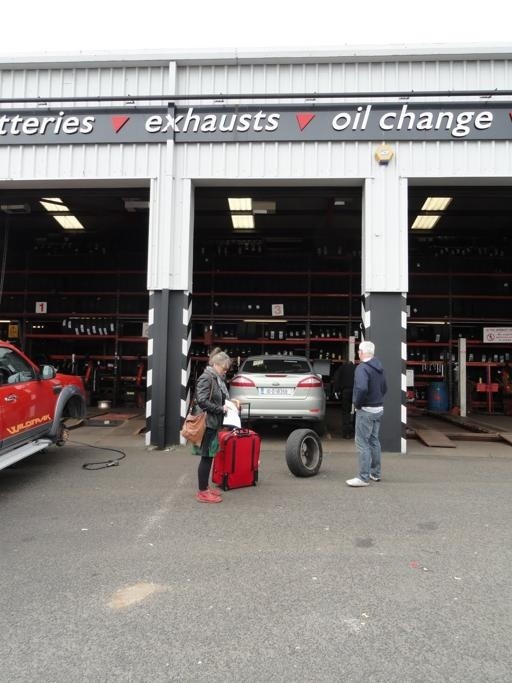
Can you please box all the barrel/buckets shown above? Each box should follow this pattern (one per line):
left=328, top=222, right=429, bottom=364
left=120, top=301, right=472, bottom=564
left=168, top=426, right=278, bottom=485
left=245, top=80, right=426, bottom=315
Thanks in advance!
left=427, top=381, right=450, bottom=411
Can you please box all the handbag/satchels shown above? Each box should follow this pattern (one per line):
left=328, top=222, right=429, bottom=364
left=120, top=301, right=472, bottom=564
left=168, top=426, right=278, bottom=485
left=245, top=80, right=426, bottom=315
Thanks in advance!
left=179, top=410, right=209, bottom=448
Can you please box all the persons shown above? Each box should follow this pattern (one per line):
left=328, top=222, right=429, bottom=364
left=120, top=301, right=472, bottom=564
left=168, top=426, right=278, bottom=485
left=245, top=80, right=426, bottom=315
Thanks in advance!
left=343, top=339, right=388, bottom=486
left=192, top=346, right=240, bottom=503
left=333, top=361, right=354, bottom=439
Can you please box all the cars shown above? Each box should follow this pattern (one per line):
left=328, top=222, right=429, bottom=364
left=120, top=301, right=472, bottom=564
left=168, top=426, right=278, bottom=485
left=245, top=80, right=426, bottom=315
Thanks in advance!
left=0, top=340, right=87, bottom=471
left=229, top=354, right=327, bottom=425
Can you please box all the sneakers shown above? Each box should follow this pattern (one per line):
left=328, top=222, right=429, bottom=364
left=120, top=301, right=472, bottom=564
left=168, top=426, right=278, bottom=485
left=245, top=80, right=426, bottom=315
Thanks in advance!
left=194, top=486, right=224, bottom=503
left=346, top=477, right=370, bottom=486
left=369, top=473, right=382, bottom=481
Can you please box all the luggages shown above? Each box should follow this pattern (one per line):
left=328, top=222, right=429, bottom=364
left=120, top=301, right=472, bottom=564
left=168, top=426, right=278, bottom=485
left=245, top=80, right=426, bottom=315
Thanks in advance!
left=211, top=402, right=262, bottom=491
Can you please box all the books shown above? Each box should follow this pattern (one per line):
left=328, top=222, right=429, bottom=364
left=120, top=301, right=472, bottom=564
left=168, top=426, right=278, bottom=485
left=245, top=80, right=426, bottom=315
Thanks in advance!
left=222, top=398, right=241, bottom=428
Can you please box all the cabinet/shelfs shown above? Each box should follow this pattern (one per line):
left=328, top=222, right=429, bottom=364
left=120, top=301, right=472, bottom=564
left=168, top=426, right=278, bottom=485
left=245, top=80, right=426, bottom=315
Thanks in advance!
left=0, top=233, right=512, bottom=415
